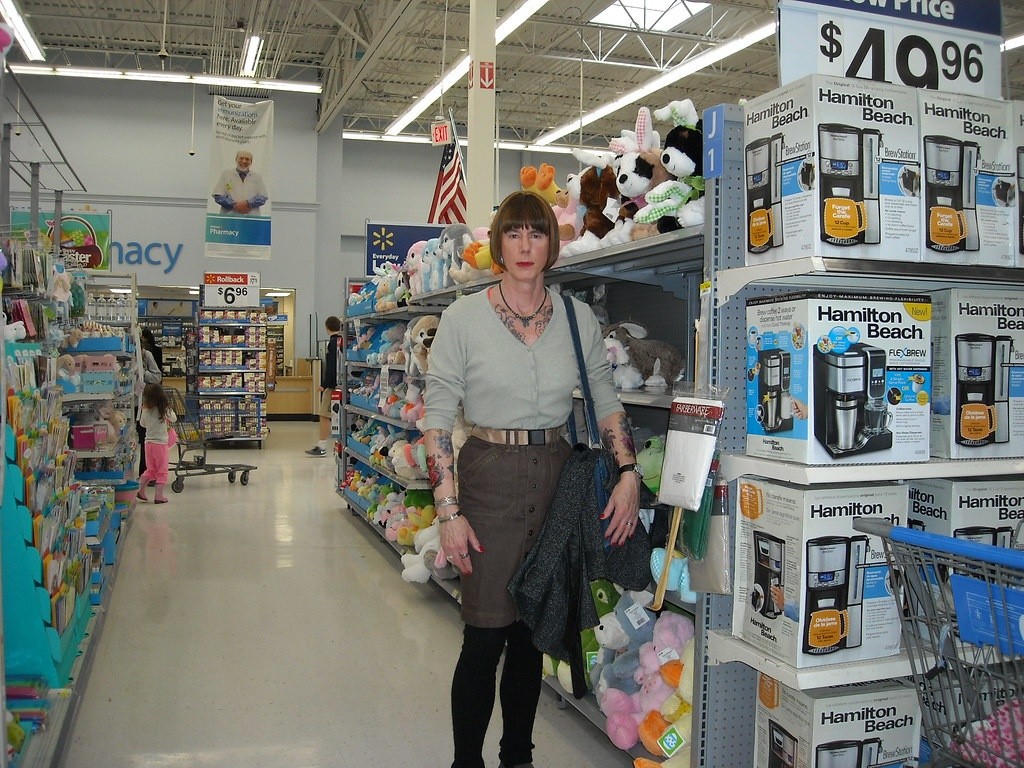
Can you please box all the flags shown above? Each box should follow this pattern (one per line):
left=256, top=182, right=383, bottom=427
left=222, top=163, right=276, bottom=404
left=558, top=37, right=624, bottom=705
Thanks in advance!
left=427, top=140, right=467, bottom=225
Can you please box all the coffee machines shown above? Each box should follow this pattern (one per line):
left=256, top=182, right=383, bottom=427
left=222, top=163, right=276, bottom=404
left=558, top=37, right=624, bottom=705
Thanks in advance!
left=744, top=137, right=771, bottom=255
left=758, top=348, right=794, bottom=433
left=954, top=334, right=995, bottom=447
left=816, top=738, right=883, bottom=768
left=1016, top=145, right=1024, bottom=254
left=767, top=719, right=799, bottom=768
left=813, top=343, right=892, bottom=457
left=751, top=530, right=786, bottom=620
left=954, top=527, right=1012, bottom=589
left=817, top=124, right=865, bottom=245
left=924, top=134, right=966, bottom=252
left=801, top=536, right=867, bottom=656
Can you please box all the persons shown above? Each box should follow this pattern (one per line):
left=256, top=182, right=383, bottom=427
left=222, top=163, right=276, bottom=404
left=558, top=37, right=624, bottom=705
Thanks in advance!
left=135, top=383, right=178, bottom=504
left=298, top=315, right=343, bottom=455
left=136, top=326, right=163, bottom=486
left=212, top=150, right=271, bottom=215
left=418, top=191, right=637, bottom=768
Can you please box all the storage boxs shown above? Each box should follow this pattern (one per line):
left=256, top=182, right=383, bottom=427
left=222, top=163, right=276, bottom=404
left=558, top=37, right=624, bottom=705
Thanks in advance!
left=91, top=574, right=109, bottom=606
left=1012, top=99, right=1024, bottom=254
left=346, top=321, right=400, bottom=363
left=238, top=416, right=268, bottom=438
left=742, top=289, right=933, bottom=467
left=91, top=559, right=106, bottom=584
left=333, top=442, right=344, bottom=489
left=906, top=658, right=1024, bottom=764
left=200, top=416, right=236, bottom=436
left=201, top=308, right=267, bottom=325
left=198, top=325, right=266, bottom=348
left=71, top=425, right=95, bottom=449
left=919, top=85, right=1017, bottom=262
left=199, top=394, right=267, bottom=415
left=346, top=419, right=419, bottom=460
left=349, top=367, right=381, bottom=413
left=330, top=390, right=342, bottom=434
left=86, top=503, right=107, bottom=537
left=739, top=72, right=921, bottom=261
left=899, top=475, right=1024, bottom=648
left=92, top=424, right=108, bottom=451
left=347, top=278, right=378, bottom=318
left=917, top=287, right=1024, bottom=461
left=83, top=371, right=118, bottom=393
left=754, top=670, right=922, bottom=768
left=66, top=336, right=123, bottom=351
left=345, top=460, right=402, bottom=512
left=729, top=474, right=912, bottom=668
left=199, top=350, right=266, bottom=370
left=76, top=468, right=126, bottom=480
left=198, top=373, right=265, bottom=392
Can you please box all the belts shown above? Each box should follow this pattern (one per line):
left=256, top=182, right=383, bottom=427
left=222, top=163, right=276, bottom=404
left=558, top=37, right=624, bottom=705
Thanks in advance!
left=470, top=427, right=562, bottom=446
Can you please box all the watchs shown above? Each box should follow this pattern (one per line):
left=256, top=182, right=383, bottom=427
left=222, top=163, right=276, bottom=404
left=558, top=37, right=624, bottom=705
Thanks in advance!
left=620, top=464, right=645, bottom=480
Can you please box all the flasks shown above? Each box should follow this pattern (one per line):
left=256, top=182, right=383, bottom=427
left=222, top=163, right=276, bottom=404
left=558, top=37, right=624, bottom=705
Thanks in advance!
left=834, top=396, right=857, bottom=450
left=767, top=391, right=778, bottom=428
left=770, top=577, right=782, bottom=615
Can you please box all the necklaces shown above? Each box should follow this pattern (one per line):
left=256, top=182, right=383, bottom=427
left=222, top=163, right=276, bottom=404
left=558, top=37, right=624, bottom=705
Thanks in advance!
left=498, top=283, right=547, bottom=328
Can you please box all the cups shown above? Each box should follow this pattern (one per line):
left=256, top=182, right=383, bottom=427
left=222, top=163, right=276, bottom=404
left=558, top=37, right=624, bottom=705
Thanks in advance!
left=864, top=401, right=893, bottom=435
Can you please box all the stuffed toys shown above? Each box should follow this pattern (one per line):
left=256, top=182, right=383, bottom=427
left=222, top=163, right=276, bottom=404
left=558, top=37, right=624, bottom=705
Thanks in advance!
left=347, top=415, right=433, bottom=480
left=520, top=98, right=706, bottom=258
left=341, top=461, right=460, bottom=586
left=100, top=410, right=128, bottom=447
left=350, top=315, right=441, bottom=377
left=416, top=406, right=474, bottom=476
left=346, top=368, right=430, bottom=432
left=541, top=578, right=695, bottom=768
left=4, top=321, right=27, bottom=343
left=347, top=224, right=506, bottom=313
left=604, top=319, right=686, bottom=388
left=633, top=430, right=697, bottom=604
left=55, top=353, right=75, bottom=380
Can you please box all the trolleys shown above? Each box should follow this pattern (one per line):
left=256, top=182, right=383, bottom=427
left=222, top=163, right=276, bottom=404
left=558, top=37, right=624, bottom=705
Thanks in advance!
left=146, top=387, right=259, bottom=493
left=852, top=515, right=1024, bottom=768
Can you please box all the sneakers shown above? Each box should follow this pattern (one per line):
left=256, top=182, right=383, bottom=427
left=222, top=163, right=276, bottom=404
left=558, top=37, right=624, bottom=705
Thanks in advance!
left=306, top=446, right=326, bottom=457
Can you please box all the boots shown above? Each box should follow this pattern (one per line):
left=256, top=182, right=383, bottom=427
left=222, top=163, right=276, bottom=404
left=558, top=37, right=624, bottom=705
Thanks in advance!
left=137, top=475, right=149, bottom=500
left=154, top=484, right=168, bottom=504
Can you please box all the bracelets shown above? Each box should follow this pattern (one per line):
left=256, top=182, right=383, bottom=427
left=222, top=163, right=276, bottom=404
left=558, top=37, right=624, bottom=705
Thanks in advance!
left=438, top=510, right=462, bottom=523
left=435, top=495, right=457, bottom=507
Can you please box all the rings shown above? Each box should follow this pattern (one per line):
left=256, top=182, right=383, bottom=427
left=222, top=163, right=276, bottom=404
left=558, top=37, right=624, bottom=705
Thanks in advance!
left=461, top=553, right=469, bottom=559
left=448, top=555, right=453, bottom=560
left=627, top=522, right=633, bottom=525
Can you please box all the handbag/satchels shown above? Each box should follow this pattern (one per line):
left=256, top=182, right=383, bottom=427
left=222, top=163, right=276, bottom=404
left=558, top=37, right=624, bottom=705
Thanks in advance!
left=593, top=456, right=615, bottom=550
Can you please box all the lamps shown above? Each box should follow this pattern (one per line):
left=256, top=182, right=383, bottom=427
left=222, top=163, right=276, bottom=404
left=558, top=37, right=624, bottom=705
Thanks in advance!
left=534, top=17, right=777, bottom=154
left=237, top=35, right=264, bottom=77
left=0, top=0, right=47, bottom=62
left=384, top=0, right=548, bottom=137
left=342, top=129, right=616, bottom=156
left=8, top=59, right=323, bottom=95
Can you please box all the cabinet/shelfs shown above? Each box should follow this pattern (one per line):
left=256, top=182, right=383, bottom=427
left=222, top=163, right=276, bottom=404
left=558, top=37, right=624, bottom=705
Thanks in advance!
left=344, top=102, right=1024, bottom=768
left=268, top=325, right=286, bottom=376
left=197, top=306, right=268, bottom=450
left=61, top=271, right=140, bottom=488
